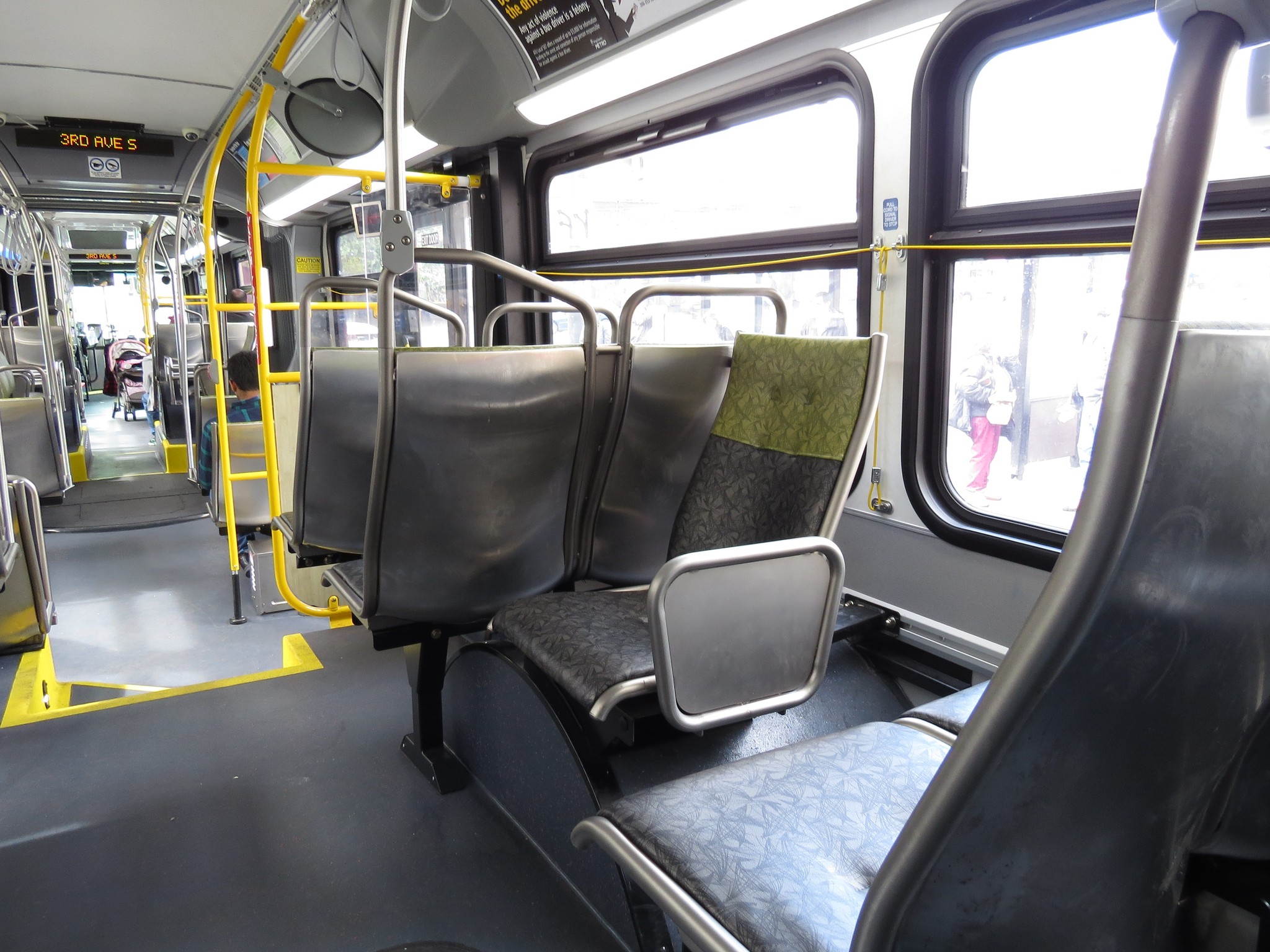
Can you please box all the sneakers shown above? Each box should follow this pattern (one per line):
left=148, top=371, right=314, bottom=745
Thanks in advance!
left=976, top=487, right=1002, bottom=500
left=240, top=555, right=251, bottom=578
left=960, top=488, right=989, bottom=508
left=148, top=437, right=157, bottom=446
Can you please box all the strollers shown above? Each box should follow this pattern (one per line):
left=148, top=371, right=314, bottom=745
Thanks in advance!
left=107, top=339, right=151, bottom=422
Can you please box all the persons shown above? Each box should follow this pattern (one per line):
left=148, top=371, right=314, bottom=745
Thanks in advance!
left=954, top=328, right=1019, bottom=497
left=198, top=351, right=282, bottom=579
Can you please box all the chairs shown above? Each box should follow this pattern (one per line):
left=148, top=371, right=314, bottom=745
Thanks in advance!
left=0, top=300, right=306, bottom=656
left=570, top=1, right=1270, bottom=952
left=319, top=247, right=597, bottom=797
left=270, top=276, right=465, bottom=568
left=482, top=302, right=621, bottom=352
left=487, top=331, right=888, bottom=733
left=572, top=287, right=788, bottom=591
